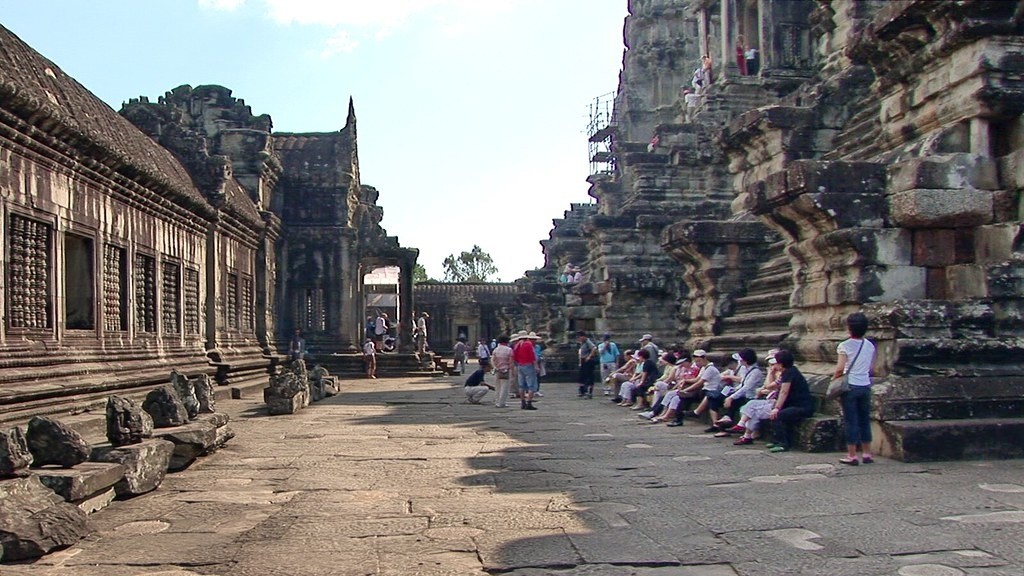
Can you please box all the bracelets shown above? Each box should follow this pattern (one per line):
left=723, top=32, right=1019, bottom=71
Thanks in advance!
left=774, top=407, right=780, bottom=410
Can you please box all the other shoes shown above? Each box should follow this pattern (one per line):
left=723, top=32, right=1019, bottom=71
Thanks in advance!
left=682, top=410, right=789, bottom=452
left=495, top=401, right=509, bottom=407
left=839, top=457, right=873, bottom=466
left=368, top=376, right=376, bottom=379
left=577, top=393, right=593, bottom=399
left=465, top=399, right=481, bottom=404
left=612, top=396, right=683, bottom=427
left=509, top=392, right=544, bottom=398
left=679, top=391, right=693, bottom=398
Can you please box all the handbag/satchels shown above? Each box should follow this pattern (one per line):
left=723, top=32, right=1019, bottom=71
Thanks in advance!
left=497, top=368, right=509, bottom=379
left=826, top=371, right=852, bottom=400
left=766, top=391, right=776, bottom=399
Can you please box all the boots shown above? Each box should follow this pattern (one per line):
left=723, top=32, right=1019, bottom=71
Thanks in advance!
left=521, top=400, right=537, bottom=410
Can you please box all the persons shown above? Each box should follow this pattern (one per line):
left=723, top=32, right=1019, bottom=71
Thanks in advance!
left=598, top=334, right=814, bottom=451
left=560, top=263, right=582, bottom=283
left=412, top=311, right=429, bottom=361
left=478, top=330, right=546, bottom=411
left=364, top=339, right=377, bottom=379
left=576, top=330, right=597, bottom=399
left=290, top=327, right=305, bottom=361
left=678, top=56, right=712, bottom=123
left=453, top=338, right=471, bottom=374
left=736, top=34, right=759, bottom=76
left=366, top=313, right=391, bottom=353
left=831, top=312, right=875, bottom=466
left=647, top=135, right=660, bottom=153
left=463, top=362, right=497, bottom=405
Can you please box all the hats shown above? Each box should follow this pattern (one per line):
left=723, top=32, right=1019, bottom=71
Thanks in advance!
left=422, top=312, right=429, bottom=317
left=766, top=349, right=794, bottom=364
left=630, top=350, right=639, bottom=359
left=567, top=263, right=581, bottom=272
left=492, top=339, right=496, bottom=341
left=658, top=350, right=706, bottom=365
left=498, top=330, right=541, bottom=342
left=732, top=348, right=755, bottom=360
left=603, top=334, right=611, bottom=338
left=639, top=335, right=651, bottom=341
